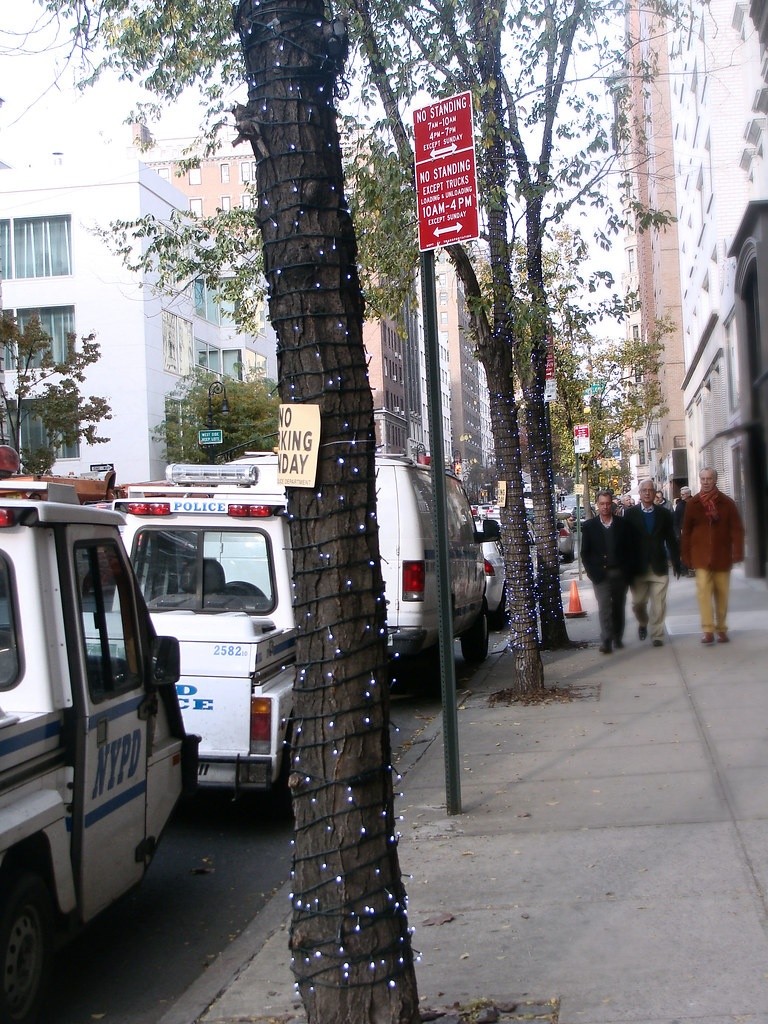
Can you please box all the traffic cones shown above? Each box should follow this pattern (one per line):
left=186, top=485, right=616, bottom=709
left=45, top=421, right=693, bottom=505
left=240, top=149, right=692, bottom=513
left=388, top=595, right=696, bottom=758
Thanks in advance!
left=564, top=579, right=589, bottom=618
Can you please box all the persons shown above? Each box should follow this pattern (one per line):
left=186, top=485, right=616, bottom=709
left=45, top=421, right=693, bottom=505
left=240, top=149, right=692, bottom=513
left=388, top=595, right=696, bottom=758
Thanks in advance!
left=677, top=485, right=702, bottom=578
left=685, top=467, right=747, bottom=644
left=581, top=491, right=636, bottom=654
left=625, top=478, right=684, bottom=647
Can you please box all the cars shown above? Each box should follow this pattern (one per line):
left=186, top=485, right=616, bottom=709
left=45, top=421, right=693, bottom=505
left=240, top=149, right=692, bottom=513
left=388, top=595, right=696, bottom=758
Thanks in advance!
left=467, top=482, right=604, bottom=629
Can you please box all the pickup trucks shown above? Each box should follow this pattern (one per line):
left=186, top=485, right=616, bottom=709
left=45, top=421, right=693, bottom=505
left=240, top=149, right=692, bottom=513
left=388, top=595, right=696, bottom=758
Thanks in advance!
left=0, top=442, right=208, bottom=1024
left=83, top=461, right=301, bottom=816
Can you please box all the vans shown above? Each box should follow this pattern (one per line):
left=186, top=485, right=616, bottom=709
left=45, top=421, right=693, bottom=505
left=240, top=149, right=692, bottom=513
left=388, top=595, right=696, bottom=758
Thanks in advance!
left=213, top=452, right=504, bottom=697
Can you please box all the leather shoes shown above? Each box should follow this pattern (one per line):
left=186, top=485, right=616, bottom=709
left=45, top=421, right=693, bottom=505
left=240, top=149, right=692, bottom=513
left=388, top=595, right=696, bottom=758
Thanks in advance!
left=717, top=632, right=729, bottom=643
left=639, top=626, right=647, bottom=640
left=599, top=641, right=611, bottom=652
left=652, top=640, right=662, bottom=646
left=701, top=632, right=714, bottom=642
left=614, top=640, right=623, bottom=648
